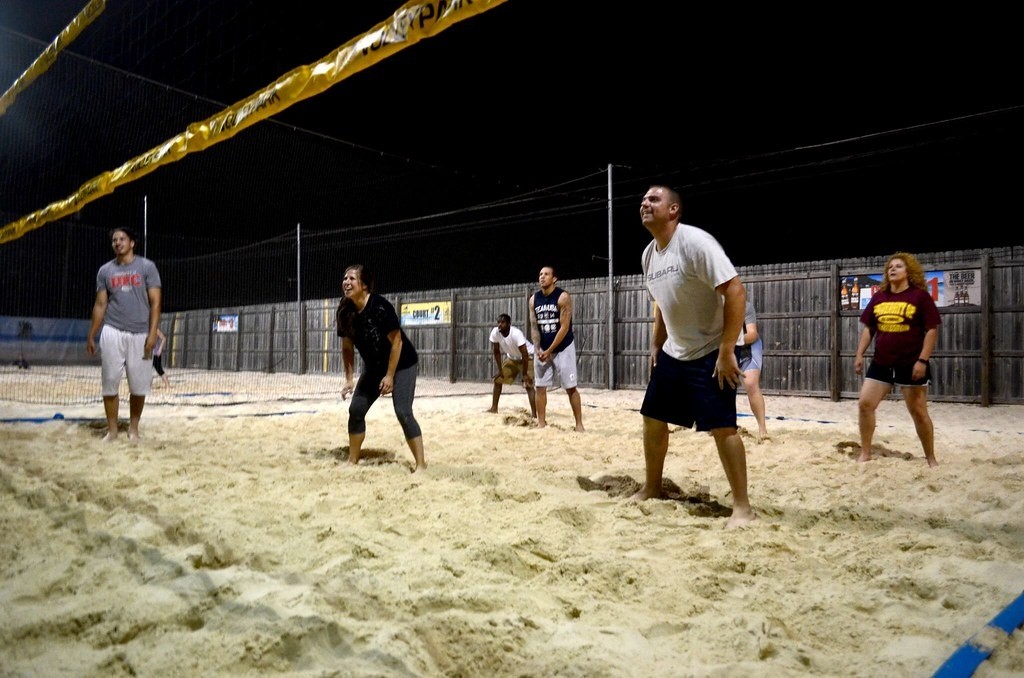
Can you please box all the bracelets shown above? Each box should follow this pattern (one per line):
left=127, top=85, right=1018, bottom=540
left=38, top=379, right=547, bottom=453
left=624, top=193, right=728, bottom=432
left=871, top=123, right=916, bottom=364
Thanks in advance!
left=918, top=358, right=927, bottom=365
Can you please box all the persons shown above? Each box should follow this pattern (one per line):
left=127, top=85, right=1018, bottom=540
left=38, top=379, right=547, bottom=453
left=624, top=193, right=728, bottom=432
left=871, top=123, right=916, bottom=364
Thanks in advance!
left=528, top=267, right=584, bottom=432
left=221, top=319, right=234, bottom=330
left=640, top=186, right=755, bottom=519
left=733, top=301, right=770, bottom=439
left=953, top=285, right=969, bottom=304
left=486, top=314, right=537, bottom=418
left=336, top=266, right=426, bottom=472
left=153, top=329, right=170, bottom=393
left=854, top=253, right=939, bottom=467
left=87, top=227, right=162, bottom=441
